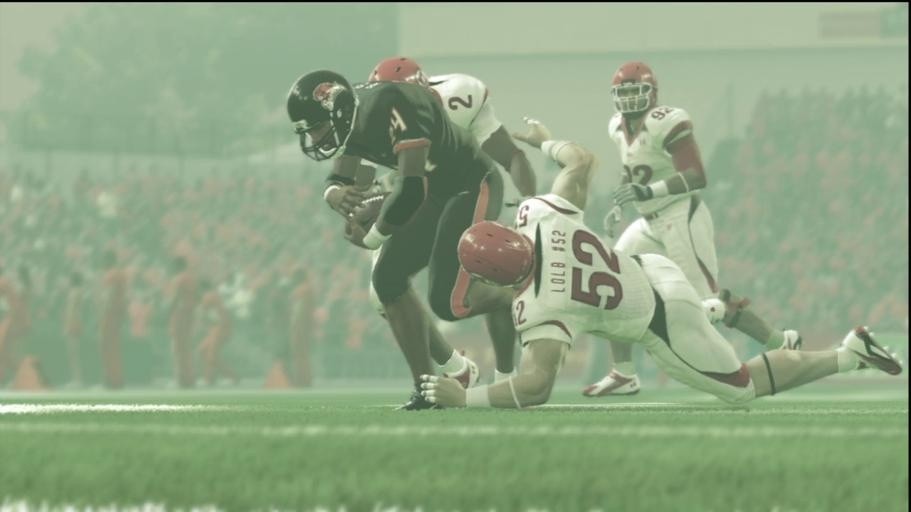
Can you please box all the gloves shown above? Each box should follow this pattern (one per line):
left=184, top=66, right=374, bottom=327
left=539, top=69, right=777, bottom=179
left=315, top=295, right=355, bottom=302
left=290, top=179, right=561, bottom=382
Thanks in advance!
left=613, top=183, right=654, bottom=206
left=604, top=206, right=622, bottom=239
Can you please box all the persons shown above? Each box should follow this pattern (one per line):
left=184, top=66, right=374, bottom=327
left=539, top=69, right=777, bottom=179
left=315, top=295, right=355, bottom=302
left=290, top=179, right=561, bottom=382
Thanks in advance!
left=1, top=152, right=409, bottom=386
left=290, top=56, right=910, bottom=405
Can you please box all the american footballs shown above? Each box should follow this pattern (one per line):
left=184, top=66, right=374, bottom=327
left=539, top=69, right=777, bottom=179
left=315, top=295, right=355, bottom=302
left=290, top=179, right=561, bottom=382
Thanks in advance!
left=343, top=192, right=393, bottom=237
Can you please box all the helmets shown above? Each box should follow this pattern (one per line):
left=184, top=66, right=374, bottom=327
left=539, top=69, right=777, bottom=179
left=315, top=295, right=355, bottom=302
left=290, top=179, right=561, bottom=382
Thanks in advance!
left=456, top=220, right=536, bottom=286
left=367, top=56, right=431, bottom=85
left=285, top=70, right=356, bottom=137
left=612, top=61, right=658, bottom=108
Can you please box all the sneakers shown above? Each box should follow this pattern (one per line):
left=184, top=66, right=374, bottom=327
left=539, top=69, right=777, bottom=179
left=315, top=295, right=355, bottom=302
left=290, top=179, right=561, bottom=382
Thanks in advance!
left=443, top=354, right=482, bottom=389
left=843, top=326, right=904, bottom=376
left=394, top=387, right=446, bottom=410
left=718, top=290, right=772, bottom=345
left=774, top=329, right=803, bottom=351
left=581, top=369, right=642, bottom=396
